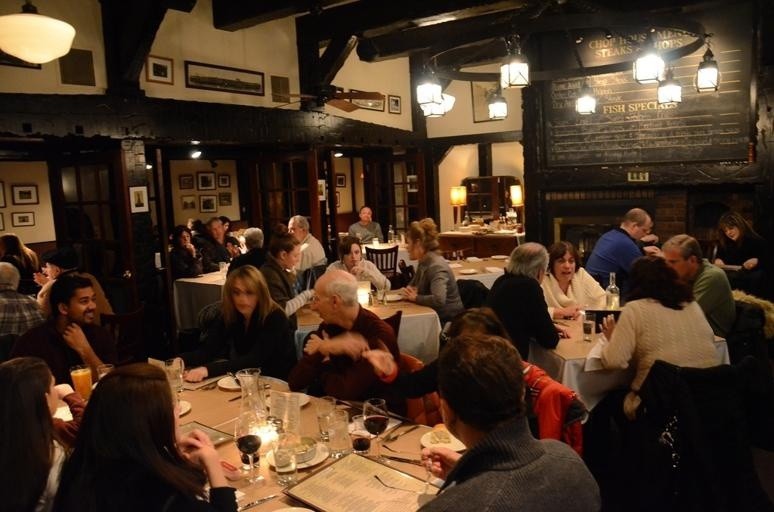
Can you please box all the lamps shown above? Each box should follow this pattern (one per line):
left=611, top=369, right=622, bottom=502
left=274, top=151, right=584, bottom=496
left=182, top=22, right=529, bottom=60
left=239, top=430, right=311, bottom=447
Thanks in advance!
left=575, top=84, right=596, bottom=116
left=695, top=49, right=720, bottom=91
left=511, top=183, right=521, bottom=207
left=415, top=68, right=442, bottom=105
left=187, top=150, right=202, bottom=158
left=487, top=91, right=507, bottom=118
left=333, top=151, right=342, bottom=158
left=659, top=71, right=683, bottom=107
left=190, top=140, right=201, bottom=146
left=426, top=62, right=459, bottom=119
left=0, top=1, right=74, bottom=63
left=502, top=36, right=531, bottom=89
left=633, top=34, right=664, bottom=84
left=450, top=185, right=466, bottom=205
left=145, top=163, right=152, bottom=170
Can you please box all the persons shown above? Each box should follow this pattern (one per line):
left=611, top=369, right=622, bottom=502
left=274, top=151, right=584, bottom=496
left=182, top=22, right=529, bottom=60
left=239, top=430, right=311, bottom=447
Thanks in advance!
left=52, top=364, right=239, bottom=512
left=363, top=308, right=540, bottom=440
left=169, top=225, right=201, bottom=277
left=398, top=218, right=464, bottom=319
left=185, top=265, right=297, bottom=382
left=349, top=205, right=384, bottom=243
left=220, top=216, right=230, bottom=231
left=187, top=218, right=202, bottom=236
left=639, top=213, right=659, bottom=245
left=287, top=214, right=327, bottom=296
left=715, top=210, right=773, bottom=271
left=260, top=224, right=316, bottom=317
left=324, top=236, right=391, bottom=292
left=0, top=233, right=42, bottom=296
left=486, top=242, right=560, bottom=361
left=10, top=275, right=105, bottom=384
left=0, top=261, right=46, bottom=337
left=600, top=257, right=730, bottom=419
left=204, top=217, right=241, bottom=272
left=52, top=384, right=86, bottom=448
left=227, top=227, right=272, bottom=275
left=661, top=234, right=735, bottom=339
left=539, top=242, right=607, bottom=320
left=33, top=247, right=112, bottom=326
left=586, top=208, right=651, bottom=289
left=0, top=358, right=66, bottom=512
left=288, top=269, right=399, bottom=414
left=415, top=333, right=601, bottom=512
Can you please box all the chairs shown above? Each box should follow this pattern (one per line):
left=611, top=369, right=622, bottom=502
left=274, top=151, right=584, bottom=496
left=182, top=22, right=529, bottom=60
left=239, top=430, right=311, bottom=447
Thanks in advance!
left=364, top=245, right=401, bottom=289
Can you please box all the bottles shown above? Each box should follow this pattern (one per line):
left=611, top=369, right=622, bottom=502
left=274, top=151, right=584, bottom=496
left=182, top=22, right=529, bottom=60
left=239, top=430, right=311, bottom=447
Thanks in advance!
left=606, top=273, right=620, bottom=310
left=388, top=225, right=394, bottom=245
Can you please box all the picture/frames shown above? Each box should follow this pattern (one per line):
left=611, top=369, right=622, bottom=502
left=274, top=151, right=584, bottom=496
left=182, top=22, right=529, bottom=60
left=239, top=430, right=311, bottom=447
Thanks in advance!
left=0, top=213, right=3, bottom=232
left=179, top=173, right=193, bottom=190
left=0, top=181, right=7, bottom=208
left=10, top=211, right=35, bottom=227
left=349, top=88, right=385, bottom=111
left=180, top=194, right=196, bottom=209
left=11, top=183, right=39, bottom=205
left=182, top=60, right=265, bottom=98
left=219, top=191, right=232, bottom=206
left=335, top=175, right=347, bottom=188
left=128, top=185, right=150, bottom=214
left=146, top=54, right=174, bottom=84
left=218, top=174, right=232, bottom=189
left=196, top=171, right=217, bottom=191
left=388, top=94, right=400, bottom=115
left=327, top=192, right=340, bottom=207
left=198, top=194, right=218, bottom=214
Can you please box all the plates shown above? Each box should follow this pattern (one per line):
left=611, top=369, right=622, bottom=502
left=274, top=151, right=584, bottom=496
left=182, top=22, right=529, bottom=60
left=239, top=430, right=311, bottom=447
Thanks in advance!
left=421, top=432, right=466, bottom=451
left=267, top=442, right=330, bottom=470
left=393, top=235, right=398, bottom=244
left=492, top=255, right=508, bottom=259
left=219, top=262, right=226, bottom=274
left=460, top=269, right=477, bottom=274
left=486, top=267, right=504, bottom=273
left=385, top=294, right=403, bottom=301
left=265, top=392, right=309, bottom=408
left=178, top=401, right=192, bottom=415
left=217, top=376, right=243, bottom=390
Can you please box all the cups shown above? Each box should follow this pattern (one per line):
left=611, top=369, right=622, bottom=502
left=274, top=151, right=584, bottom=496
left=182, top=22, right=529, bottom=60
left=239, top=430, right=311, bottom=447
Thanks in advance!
left=352, top=415, right=367, bottom=430
left=69, top=363, right=93, bottom=400
left=96, top=363, right=113, bottom=377
left=327, top=410, right=349, bottom=457
left=273, top=443, right=299, bottom=486
left=316, top=396, right=336, bottom=441
left=582, top=313, right=595, bottom=341
left=234, top=418, right=265, bottom=488
left=363, top=397, right=391, bottom=465
left=350, top=430, right=371, bottom=454
left=166, top=360, right=183, bottom=398
